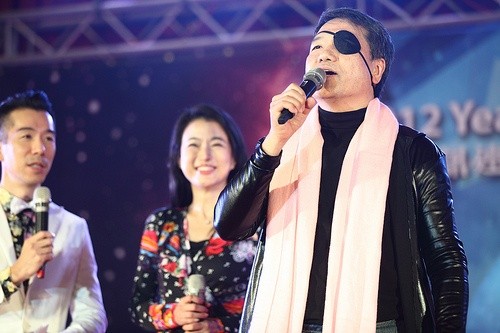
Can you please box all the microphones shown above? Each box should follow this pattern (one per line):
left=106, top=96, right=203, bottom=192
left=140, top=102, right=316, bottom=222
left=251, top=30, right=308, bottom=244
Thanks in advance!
left=278, top=68, right=327, bottom=125
left=188, top=274, right=206, bottom=323
left=33, top=187, right=52, bottom=279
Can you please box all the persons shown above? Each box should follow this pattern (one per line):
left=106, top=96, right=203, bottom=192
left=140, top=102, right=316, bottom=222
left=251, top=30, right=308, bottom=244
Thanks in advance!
left=0, top=91, right=108, bottom=333
left=130, top=102, right=267, bottom=333
left=215, top=10, right=469, bottom=333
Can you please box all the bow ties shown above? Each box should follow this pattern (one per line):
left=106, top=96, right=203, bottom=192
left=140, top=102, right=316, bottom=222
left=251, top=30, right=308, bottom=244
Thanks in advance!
left=11, top=198, right=37, bottom=214
